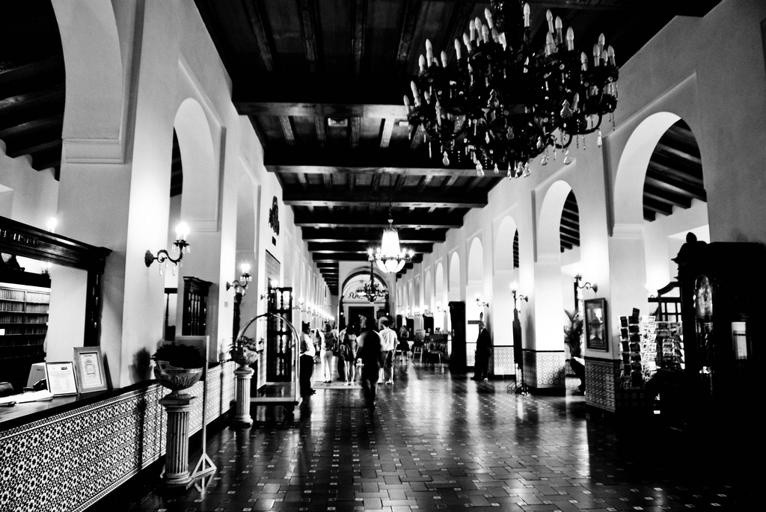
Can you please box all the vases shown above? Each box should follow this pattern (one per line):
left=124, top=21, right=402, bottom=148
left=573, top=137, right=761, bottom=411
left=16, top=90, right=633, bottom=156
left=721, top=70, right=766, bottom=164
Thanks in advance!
left=155, top=367, right=203, bottom=402
left=231, top=352, right=258, bottom=372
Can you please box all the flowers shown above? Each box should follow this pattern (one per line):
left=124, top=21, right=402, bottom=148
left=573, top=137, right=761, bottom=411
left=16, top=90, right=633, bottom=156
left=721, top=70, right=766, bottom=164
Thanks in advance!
left=227, top=337, right=259, bottom=355
left=151, top=343, right=203, bottom=370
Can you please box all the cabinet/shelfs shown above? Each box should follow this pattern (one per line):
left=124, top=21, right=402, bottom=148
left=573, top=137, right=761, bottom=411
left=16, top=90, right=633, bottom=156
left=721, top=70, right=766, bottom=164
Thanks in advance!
left=0, top=286, right=50, bottom=344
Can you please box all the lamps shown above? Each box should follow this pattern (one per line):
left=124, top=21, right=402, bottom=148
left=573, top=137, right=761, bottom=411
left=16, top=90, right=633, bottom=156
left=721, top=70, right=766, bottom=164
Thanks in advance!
left=365, top=172, right=415, bottom=275
left=226, top=261, right=251, bottom=290
left=403, top=0, right=618, bottom=178
left=145, top=222, right=191, bottom=267
left=406, top=263, right=598, bottom=318
left=356, top=262, right=389, bottom=304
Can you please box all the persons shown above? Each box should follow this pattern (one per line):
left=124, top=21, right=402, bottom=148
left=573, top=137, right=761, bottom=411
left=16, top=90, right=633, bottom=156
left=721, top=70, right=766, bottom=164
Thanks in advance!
left=311, top=315, right=416, bottom=410
left=470, top=322, right=492, bottom=380
left=299, top=321, right=316, bottom=398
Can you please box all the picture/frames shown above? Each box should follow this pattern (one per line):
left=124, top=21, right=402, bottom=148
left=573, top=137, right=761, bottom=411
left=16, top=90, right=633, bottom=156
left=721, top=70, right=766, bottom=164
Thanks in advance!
left=584, top=298, right=609, bottom=351
left=73, top=346, right=108, bottom=395
left=44, top=360, right=78, bottom=397
